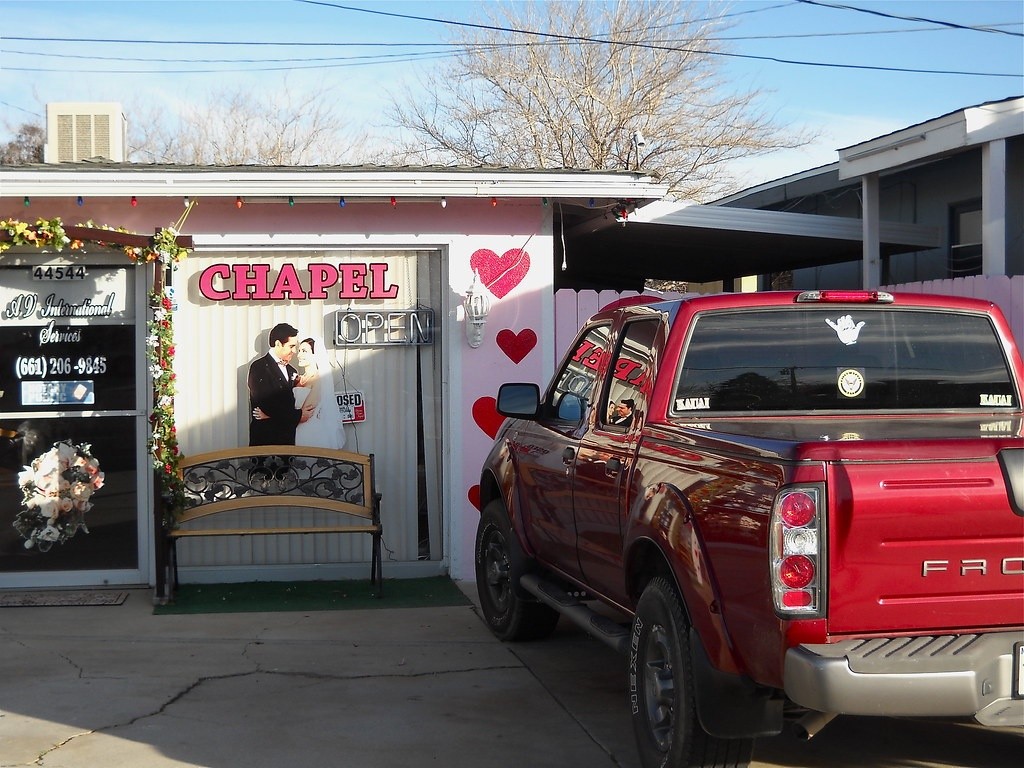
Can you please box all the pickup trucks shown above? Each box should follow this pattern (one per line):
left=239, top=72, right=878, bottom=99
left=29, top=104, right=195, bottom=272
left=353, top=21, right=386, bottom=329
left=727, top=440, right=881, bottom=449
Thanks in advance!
left=474, top=286, right=1024, bottom=768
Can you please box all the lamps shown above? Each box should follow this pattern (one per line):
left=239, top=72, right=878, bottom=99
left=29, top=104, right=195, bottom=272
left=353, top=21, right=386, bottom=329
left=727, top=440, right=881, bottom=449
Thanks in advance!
left=463, top=267, right=490, bottom=349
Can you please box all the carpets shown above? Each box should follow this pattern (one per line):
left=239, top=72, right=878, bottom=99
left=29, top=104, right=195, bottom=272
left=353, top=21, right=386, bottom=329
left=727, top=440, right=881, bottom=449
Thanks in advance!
left=0, top=590, right=130, bottom=607
left=151, top=573, right=476, bottom=616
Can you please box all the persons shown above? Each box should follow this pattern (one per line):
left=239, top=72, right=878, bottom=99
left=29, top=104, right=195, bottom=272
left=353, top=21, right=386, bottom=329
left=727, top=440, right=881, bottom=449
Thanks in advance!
left=606, top=401, right=636, bottom=427
left=243, top=321, right=346, bottom=479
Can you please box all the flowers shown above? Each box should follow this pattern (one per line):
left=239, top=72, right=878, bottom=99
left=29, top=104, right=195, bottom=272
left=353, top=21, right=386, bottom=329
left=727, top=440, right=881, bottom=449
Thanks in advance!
left=0, top=216, right=194, bottom=531
left=290, top=373, right=301, bottom=384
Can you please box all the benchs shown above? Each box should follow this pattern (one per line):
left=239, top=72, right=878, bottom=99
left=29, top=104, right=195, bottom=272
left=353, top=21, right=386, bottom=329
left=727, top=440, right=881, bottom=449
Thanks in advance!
left=165, top=444, right=384, bottom=604
